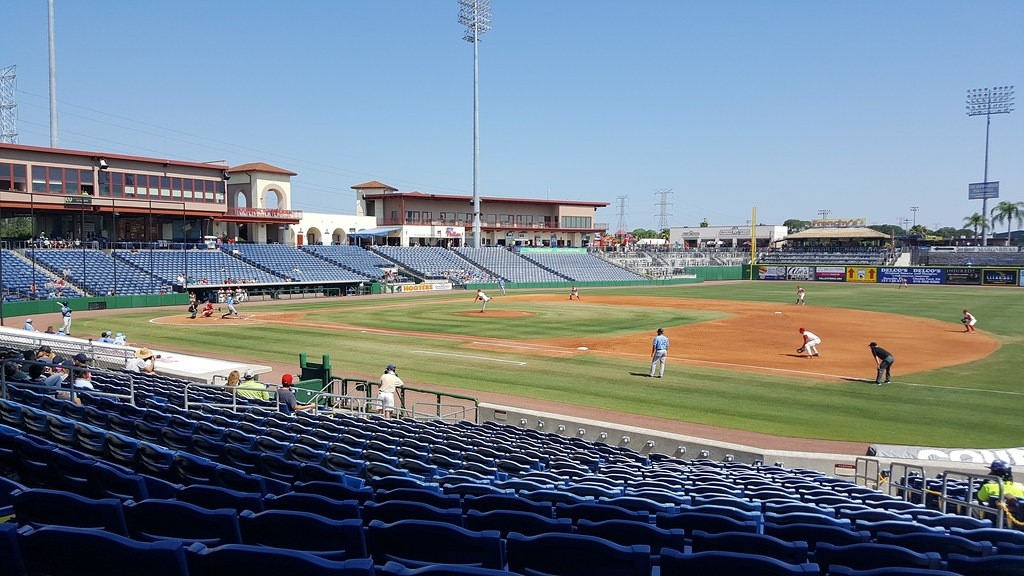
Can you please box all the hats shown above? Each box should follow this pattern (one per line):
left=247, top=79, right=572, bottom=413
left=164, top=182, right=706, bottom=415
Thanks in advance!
left=985, top=460, right=1010, bottom=472
left=137, top=347, right=153, bottom=360
left=386, top=364, right=396, bottom=370
left=116, top=333, right=122, bottom=337
left=244, top=370, right=254, bottom=379
left=52, top=356, right=66, bottom=365
left=38, top=345, right=51, bottom=354
left=282, top=374, right=293, bottom=386
left=26, top=319, right=32, bottom=322
left=799, top=327, right=805, bottom=333
left=106, top=331, right=111, bottom=335
left=59, top=328, right=64, bottom=332
left=869, top=342, right=878, bottom=347
left=657, top=329, right=664, bottom=333
left=73, top=354, right=87, bottom=362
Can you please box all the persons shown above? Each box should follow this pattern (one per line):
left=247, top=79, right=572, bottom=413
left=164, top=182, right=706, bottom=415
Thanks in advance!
left=274, top=374, right=316, bottom=412
left=126, top=347, right=156, bottom=374
left=962, top=309, right=977, bottom=333
left=237, top=369, right=269, bottom=401
left=224, top=371, right=240, bottom=394
left=897, top=277, right=907, bottom=289
left=970, top=459, right=1023, bottom=520
left=28, top=229, right=95, bottom=248
left=131, top=246, right=138, bottom=253
left=376, top=364, right=405, bottom=418
left=474, top=289, right=493, bottom=313
left=780, top=240, right=882, bottom=252
left=0, top=267, right=136, bottom=407
left=569, top=286, right=580, bottom=300
left=350, top=239, right=467, bottom=252
left=795, top=285, right=805, bottom=306
left=365, top=268, right=506, bottom=296
left=868, top=342, right=895, bottom=385
left=797, top=327, right=821, bottom=359
left=177, top=236, right=282, bottom=319
left=645, top=327, right=669, bottom=378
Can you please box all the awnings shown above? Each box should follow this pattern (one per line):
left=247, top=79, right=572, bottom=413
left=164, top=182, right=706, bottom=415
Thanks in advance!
left=347, top=226, right=401, bottom=239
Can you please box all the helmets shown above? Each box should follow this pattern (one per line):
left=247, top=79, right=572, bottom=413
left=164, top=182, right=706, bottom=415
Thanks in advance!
left=63, top=301, right=68, bottom=305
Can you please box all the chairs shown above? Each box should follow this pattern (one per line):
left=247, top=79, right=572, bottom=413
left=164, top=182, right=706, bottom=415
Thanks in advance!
left=0, top=239, right=1024, bottom=576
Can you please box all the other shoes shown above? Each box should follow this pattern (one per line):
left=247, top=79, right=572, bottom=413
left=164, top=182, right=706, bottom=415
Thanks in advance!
left=812, top=354, right=818, bottom=356
left=885, top=380, right=890, bottom=383
left=971, top=331, right=975, bottom=334
left=654, top=375, right=662, bottom=379
left=872, top=382, right=882, bottom=386
left=645, top=375, right=653, bottom=378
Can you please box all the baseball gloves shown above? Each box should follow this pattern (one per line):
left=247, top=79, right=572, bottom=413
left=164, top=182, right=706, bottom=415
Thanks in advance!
left=797, top=347, right=805, bottom=353
left=218, top=307, right=222, bottom=311
left=960, top=318, right=966, bottom=323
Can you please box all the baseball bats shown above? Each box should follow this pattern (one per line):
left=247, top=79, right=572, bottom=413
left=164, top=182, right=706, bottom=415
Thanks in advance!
left=57, top=302, right=64, bottom=306
left=237, top=301, right=248, bottom=310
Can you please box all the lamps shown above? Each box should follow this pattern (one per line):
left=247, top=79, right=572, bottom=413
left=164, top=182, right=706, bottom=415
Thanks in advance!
left=99, top=159, right=110, bottom=169
left=223, top=172, right=231, bottom=180
